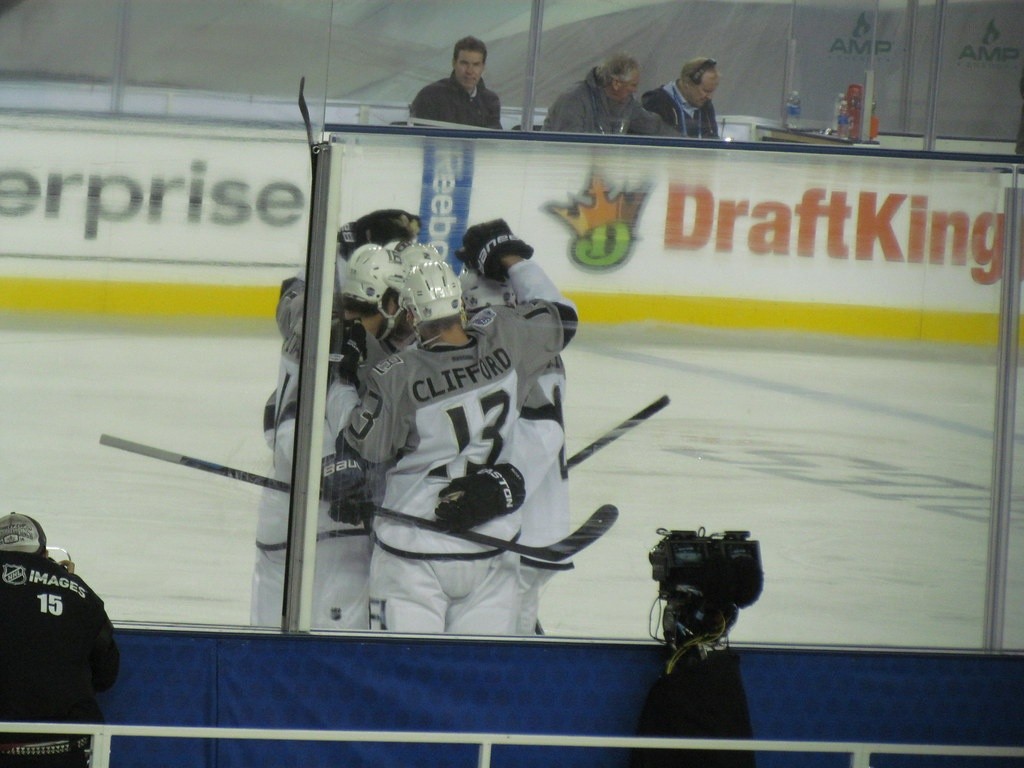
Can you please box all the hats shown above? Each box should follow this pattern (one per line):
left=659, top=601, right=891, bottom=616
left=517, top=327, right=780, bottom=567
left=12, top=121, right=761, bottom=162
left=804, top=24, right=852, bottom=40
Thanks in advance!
left=0, top=512, right=47, bottom=553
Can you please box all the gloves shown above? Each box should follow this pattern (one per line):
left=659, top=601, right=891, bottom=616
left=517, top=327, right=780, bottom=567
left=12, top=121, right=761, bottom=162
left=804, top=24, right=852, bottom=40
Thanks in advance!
left=435, top=463, right=526, bottom=532
left=332, top=321, right=367, bottom=383
left=322, top=430, right=376, bottom=526
left=337, top=208, right=421, bottom=249
left=455, top=218, right=534, bottom=284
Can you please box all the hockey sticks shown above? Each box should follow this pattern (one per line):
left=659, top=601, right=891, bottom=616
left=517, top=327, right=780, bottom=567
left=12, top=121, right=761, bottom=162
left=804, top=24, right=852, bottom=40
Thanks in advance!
left=566, top=393, right=670, bottom=473
left=97, top=432, right=620, bottom=563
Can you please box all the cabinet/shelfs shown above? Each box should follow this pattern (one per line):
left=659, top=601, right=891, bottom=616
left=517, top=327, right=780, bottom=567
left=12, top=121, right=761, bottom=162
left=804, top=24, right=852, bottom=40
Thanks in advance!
left=755, top=123, right=879, bottom=146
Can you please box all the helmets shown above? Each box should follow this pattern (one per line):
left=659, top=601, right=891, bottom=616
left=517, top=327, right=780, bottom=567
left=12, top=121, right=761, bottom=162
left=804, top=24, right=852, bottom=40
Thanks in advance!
left=458, top=263, right=517, bottom=312
left=341, top=244, right=386, bottom=307
left=383, top=241, right=462, bottom=325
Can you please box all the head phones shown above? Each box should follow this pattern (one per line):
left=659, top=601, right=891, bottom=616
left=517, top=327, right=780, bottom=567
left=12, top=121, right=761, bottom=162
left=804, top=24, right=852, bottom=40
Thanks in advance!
left=691, top=58, right=716, bottom=84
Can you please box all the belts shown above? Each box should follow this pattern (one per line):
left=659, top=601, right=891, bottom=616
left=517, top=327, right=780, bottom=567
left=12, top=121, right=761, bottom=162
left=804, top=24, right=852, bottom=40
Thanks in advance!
left=0, top=736, right=89, bottom=755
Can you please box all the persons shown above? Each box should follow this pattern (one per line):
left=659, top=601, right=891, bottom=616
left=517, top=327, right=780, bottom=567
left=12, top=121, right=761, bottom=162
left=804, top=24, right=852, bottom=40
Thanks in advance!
left=540, top=51, right=689, bottom=137
left=410, top=37, right=503, bottom=131
left=1, top=512, right=120, bottom=768
left=642, top=58, right=722, bottom=139
left=250, top=209, right=578, bottom=633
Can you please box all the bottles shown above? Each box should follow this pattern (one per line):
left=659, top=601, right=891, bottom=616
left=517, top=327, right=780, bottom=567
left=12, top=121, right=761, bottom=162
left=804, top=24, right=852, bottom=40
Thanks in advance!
left=785, top=90, right=801, bottom=128
left=833, top=94, right=846, bottom=130
left=837, top=101, right=850, bottom=139
